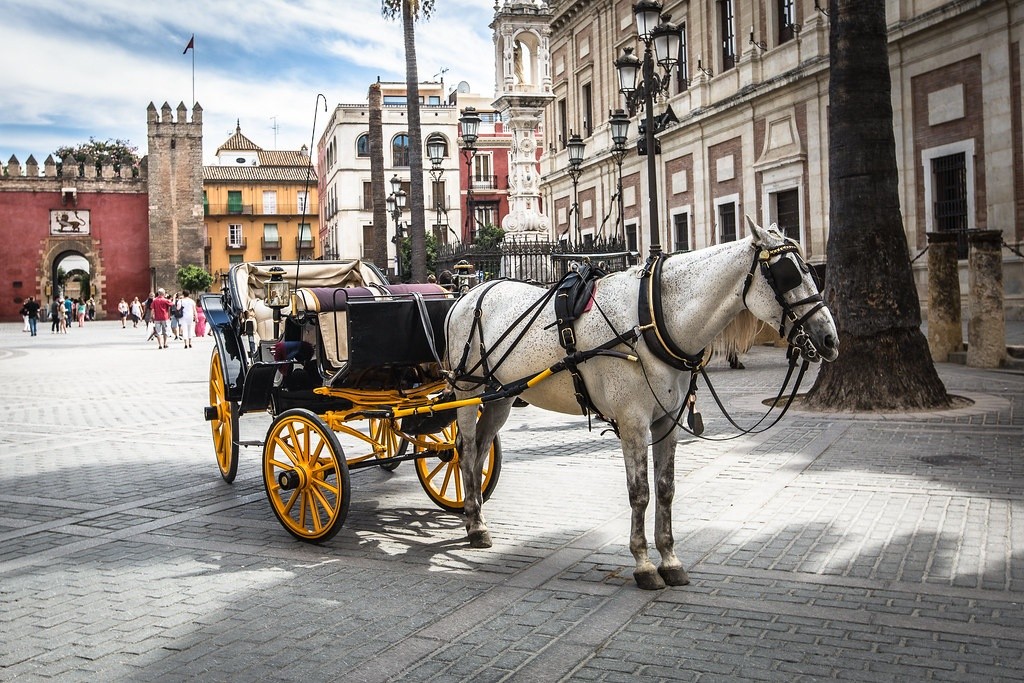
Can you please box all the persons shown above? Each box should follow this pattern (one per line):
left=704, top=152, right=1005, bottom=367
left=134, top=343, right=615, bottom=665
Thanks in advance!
left=142, top=288, right=212, bottom=349
left=20, top=296, right=39, bottom=336
left=51, top=296, right=96, bottom=334
left=129, top=297, right=143, bottom=328
left=427, top=274, right=437, bottom=284
left=117, top=298, right=130, bottom=327
left=439, top=270, right=453, bottom=291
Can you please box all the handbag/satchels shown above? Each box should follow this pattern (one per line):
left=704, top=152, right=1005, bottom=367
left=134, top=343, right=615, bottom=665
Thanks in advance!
left=174, top=306, right=184, bottom=319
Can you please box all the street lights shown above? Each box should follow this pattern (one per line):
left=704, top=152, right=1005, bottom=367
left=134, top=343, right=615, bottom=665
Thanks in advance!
left=613, top=0, right=686, bottom=262
left=608, top=109, right=631, bottom=269
left=565, top=133, right=588, bottom=254
left=427, top=134, right=448, bottom=283
left=386, top=173, right=407, bottom=284
left=458, top=104, right=482, bottom=245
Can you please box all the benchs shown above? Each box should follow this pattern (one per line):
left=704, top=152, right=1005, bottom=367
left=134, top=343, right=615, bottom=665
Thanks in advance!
left=295, top=284, right=454, bottom=377
left=236, top=288, right=296, bottom=355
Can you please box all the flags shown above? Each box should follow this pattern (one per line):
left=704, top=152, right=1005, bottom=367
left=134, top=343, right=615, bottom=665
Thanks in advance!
left=183, top=37, right=193, bottom=54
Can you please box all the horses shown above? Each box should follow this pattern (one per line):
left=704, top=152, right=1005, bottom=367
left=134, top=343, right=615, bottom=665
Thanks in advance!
left=444, top=212, right=840, bottom=590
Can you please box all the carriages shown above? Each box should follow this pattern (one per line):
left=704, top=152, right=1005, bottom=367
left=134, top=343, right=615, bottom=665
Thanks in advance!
left=195, top=211, right=842, bottom=593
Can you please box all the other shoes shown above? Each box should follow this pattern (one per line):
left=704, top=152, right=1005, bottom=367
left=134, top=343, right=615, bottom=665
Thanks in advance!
left=185, top=345, right=188, bottom=349
left=189, top=345, right=192, bottom=348
left=174, top=336, right=178, bottom=340
left=159, top=346, right=162, bottom=349
left=164, top=345, right=168, bottom=348
left=179, top=337, right=183, bottom=340
